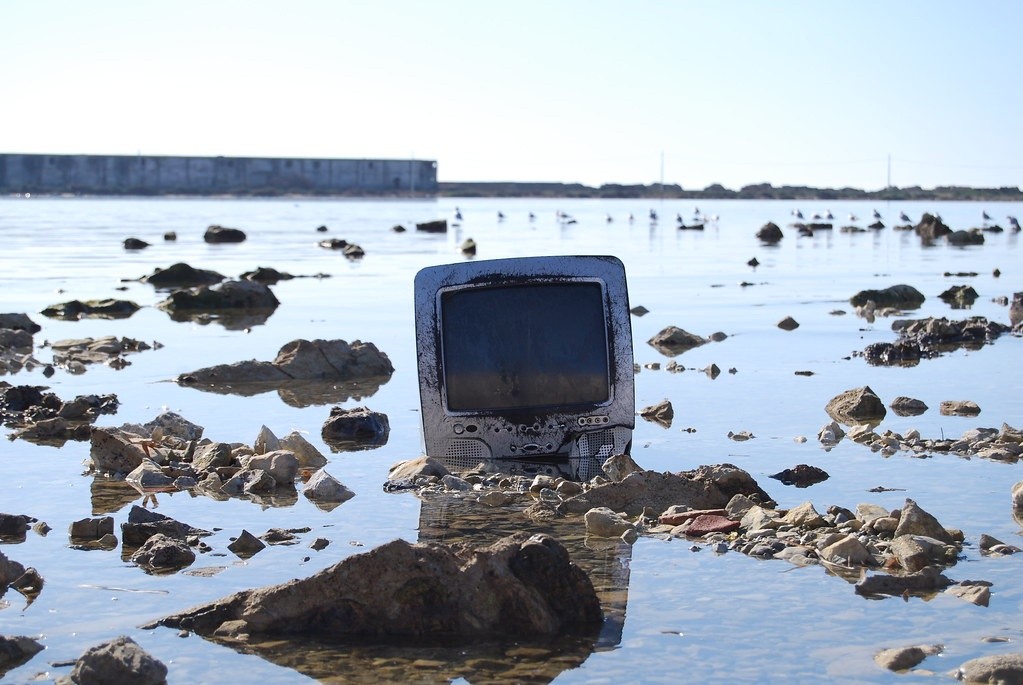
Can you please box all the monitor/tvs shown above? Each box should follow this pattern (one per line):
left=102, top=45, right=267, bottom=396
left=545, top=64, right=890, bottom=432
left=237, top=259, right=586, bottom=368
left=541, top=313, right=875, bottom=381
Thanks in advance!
left=414, top=255, right=636, bottom=460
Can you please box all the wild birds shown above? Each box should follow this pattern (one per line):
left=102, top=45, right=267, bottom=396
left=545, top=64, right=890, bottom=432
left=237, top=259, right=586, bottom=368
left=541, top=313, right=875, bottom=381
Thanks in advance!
left=451, top=202, right=1023, bottom=232
